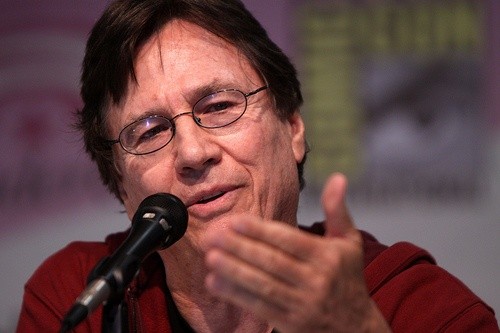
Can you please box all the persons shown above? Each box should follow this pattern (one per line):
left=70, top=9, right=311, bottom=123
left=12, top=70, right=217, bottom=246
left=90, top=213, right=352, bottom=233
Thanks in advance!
left=15, top=0, right=499, bottom=333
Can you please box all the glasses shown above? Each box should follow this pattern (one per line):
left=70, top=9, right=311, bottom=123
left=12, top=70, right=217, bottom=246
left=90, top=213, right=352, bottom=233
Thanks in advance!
left=107, top=85, right=270, bottom=155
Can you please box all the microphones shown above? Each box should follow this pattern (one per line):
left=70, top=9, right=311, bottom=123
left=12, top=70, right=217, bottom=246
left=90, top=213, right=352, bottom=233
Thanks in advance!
left=60, top=193, right=188, bottom=333
